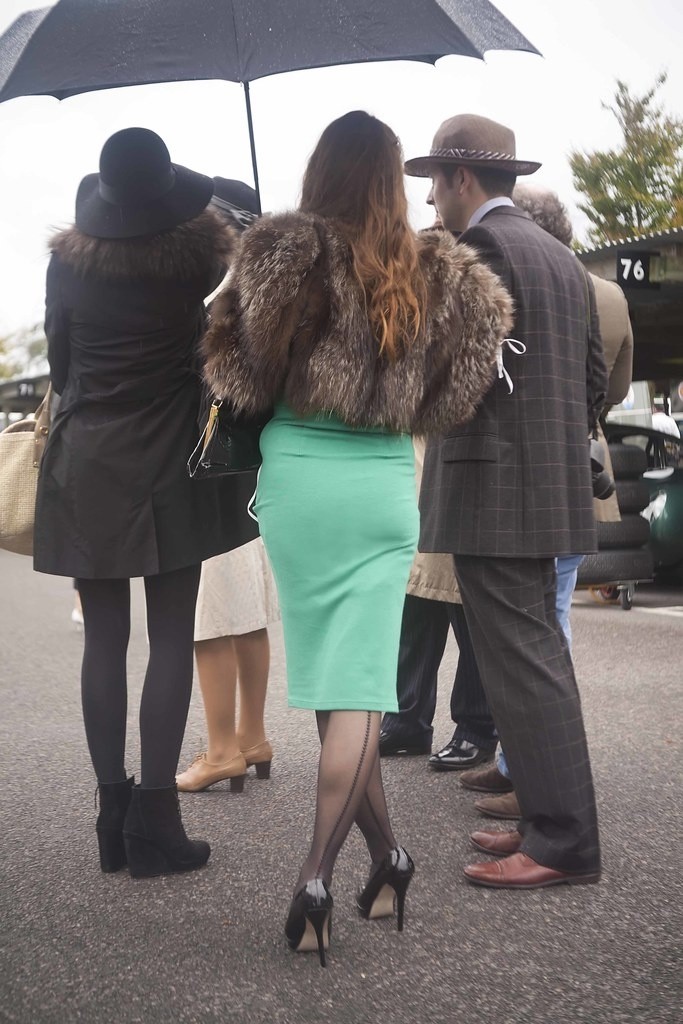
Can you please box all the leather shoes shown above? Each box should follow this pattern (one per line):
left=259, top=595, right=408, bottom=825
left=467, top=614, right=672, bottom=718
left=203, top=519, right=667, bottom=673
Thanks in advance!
left=464, top=854, right=602, bottom=888
left=429, top=738, right=496, bottom=769
left=470, top=828, right=525, bottom=855
left=378, top=731, right=434, bottom=755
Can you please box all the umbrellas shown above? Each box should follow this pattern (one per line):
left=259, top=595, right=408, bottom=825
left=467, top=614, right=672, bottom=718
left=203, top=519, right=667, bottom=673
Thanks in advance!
left=0, top=0, right=542, bottom=216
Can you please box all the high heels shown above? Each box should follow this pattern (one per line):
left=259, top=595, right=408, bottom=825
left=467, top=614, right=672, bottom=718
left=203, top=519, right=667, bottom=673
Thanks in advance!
left=122, top=782, right=210, bottom=877
left=177, top=749, right=247, bottom=794
left=94, top=776, right=136, bottom=873
left=354, top=847, right=413, bottom=930
left=285, top=879, right=333, bottom=968
left=242, top=740, right=273, bottom=780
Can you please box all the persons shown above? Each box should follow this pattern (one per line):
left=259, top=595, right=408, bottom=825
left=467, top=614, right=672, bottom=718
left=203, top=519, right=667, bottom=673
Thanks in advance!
left=171, top=178, right=281, bottom=793
left=402, top=112, right=605, bottom=889
left=376, top=185, right=631, bottom=820
left=34, top=127, right=261, bottom=878
left=203, top=109, right=513, bottom=965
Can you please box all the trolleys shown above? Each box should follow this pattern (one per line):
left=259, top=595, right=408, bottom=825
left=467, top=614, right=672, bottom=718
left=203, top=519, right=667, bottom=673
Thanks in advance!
left=571, top=576, right=661, bottom=610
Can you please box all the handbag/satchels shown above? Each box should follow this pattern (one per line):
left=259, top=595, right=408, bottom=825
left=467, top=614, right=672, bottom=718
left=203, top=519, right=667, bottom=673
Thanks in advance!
left=186, top=392, right=266, bottom=481
left=1, top=377, right=55, bottom=557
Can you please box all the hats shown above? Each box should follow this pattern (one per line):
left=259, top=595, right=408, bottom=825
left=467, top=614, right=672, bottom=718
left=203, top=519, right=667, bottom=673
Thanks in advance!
left=75, top=127, right=215, bottom=238
left=402, top=114, right=541, bottom=175
left=209, top=173, right=262, bottom=221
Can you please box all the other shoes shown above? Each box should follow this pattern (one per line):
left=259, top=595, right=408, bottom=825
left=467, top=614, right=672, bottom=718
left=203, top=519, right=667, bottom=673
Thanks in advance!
left=459, top=771, right=512, bottom=794
left=474, top=790, right=521, bottom=819
